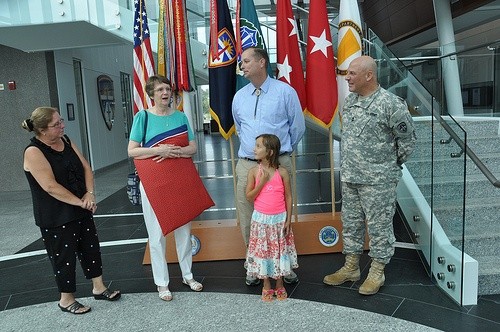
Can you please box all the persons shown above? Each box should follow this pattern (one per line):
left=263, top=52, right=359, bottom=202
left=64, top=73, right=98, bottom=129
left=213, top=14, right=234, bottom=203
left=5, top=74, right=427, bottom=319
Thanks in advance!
left=232, top=46, right=306, bottom=286
left=244, top=134, right=292, bottom=302
left=128, top=74, right=203, bottom=301
left=323, top=56, right=417, bottom=295
left=22, top=106, right=121, bottom=313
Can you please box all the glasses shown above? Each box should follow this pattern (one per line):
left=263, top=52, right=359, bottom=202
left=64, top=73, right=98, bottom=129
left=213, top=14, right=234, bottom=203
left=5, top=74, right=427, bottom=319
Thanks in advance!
left=42, top=119, right=64, bottom=128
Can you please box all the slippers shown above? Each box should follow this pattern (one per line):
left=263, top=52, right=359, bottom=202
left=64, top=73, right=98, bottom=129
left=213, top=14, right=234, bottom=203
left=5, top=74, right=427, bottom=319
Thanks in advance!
left=58, top=300, right=91, bottom=314
left=94, top=288, right=122, bottom=301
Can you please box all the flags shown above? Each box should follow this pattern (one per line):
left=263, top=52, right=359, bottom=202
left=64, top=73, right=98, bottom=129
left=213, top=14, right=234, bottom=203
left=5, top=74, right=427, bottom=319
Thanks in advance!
left=276, top=0, right=308, bottom=118
left=209, top=0, right=237, bottom=140
left=236, top=0, right=273, bottom=91
left=337, top=0, right=364, bottom=119
left=307, top=0, right=339, bottom=126
left=131, top=0, right=156, bottom=114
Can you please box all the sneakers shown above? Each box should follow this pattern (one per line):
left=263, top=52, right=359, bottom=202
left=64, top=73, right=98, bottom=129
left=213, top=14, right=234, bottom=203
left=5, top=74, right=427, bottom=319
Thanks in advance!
left=283, top=271, right=299, bottom=284
left=246, top=274, right=260, bottom=286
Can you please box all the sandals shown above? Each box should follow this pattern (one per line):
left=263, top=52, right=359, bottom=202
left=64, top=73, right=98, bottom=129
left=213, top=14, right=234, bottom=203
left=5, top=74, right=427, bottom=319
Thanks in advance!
left=157, top=286, right=172, bottom=301
left=183, top=279, right=203, bottom=291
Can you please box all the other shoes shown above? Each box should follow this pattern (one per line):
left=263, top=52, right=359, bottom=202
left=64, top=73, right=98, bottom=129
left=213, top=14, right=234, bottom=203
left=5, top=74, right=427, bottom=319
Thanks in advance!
left=274, top=287, right=287, bottom=300
left=262, top=287, right=273, bottom=302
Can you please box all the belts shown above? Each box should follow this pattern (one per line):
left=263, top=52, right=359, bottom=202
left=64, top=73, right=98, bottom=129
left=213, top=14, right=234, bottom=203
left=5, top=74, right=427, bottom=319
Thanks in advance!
left=245, top=152, right=287, bottom=162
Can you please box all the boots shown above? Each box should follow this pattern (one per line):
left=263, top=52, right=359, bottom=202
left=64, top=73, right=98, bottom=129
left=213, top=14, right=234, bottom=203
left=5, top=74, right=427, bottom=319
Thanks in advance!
left=358, top=259, right=385, bottom=295
left=323, top=254, right=360, bottom=285
